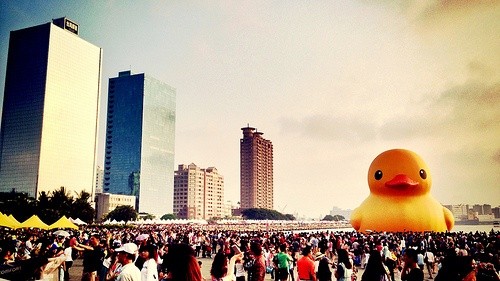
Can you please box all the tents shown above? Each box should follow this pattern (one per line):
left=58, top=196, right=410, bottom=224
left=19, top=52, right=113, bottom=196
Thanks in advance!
left=0, top=212, right=87, bottom=231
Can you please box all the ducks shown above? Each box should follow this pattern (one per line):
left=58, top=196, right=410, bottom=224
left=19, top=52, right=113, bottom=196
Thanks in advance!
left=350, top=149, right=454, bottom=235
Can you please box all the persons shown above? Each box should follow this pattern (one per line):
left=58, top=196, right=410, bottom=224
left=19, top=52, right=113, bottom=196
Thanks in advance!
left=0, top=224, right=500, bottom=281
